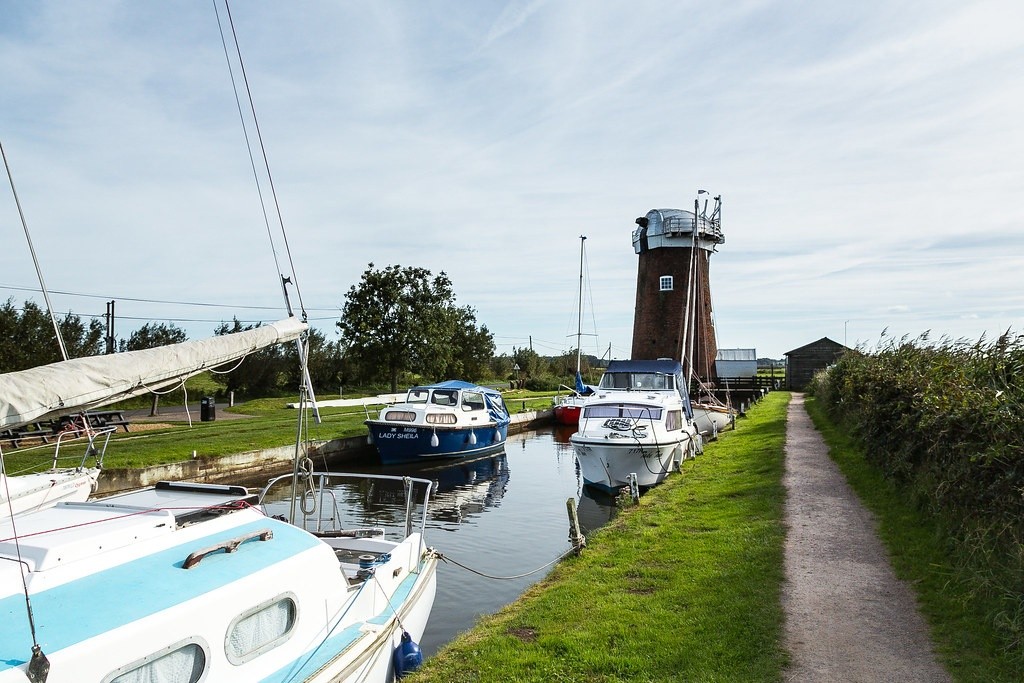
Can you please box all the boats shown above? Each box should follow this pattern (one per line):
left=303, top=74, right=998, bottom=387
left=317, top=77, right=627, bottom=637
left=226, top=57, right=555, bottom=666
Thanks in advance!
left=0, top=315, right=438, bottom=683
left=571, top=358, right=709, bottom=495
left=363, top=379, right=509, bottom=464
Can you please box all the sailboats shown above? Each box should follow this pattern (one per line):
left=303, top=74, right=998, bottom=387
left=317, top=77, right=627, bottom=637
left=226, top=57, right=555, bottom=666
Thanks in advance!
left=552, top=234, right=605, bottom=427
left=677, top=197, right=741, bottom=436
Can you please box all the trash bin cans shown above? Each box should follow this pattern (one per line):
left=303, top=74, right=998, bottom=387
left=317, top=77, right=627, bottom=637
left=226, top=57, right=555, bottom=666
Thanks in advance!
left=200, top=395, right=216, bottom=421
